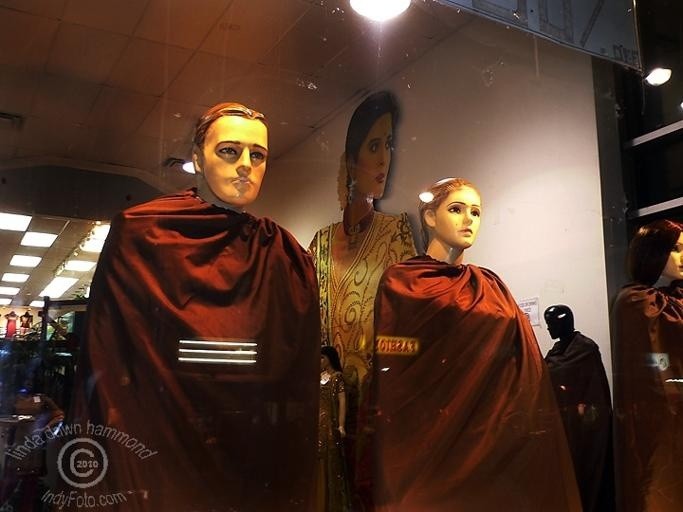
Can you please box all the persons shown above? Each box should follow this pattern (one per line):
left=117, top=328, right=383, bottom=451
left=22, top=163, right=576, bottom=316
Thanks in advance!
left=20, top=312, right=33, bottom=336
left=3, top=311, right=19, bottom=340
left=349, top=178, right=583, bottom=511
left=539, top=303, right=617, bottom=512
left=0, top=368, right=65, bottom=512
left=75, top=100, right=322, bottom=510
left=315, top=345, right=351, bottom=512
left=305, top=91, right=419, bottom=403
left=611, top=217, right=683, bottom=510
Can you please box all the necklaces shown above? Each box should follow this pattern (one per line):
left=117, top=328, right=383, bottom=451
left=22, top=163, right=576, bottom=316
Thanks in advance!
left=343, top=203, right=373, bottom=261
